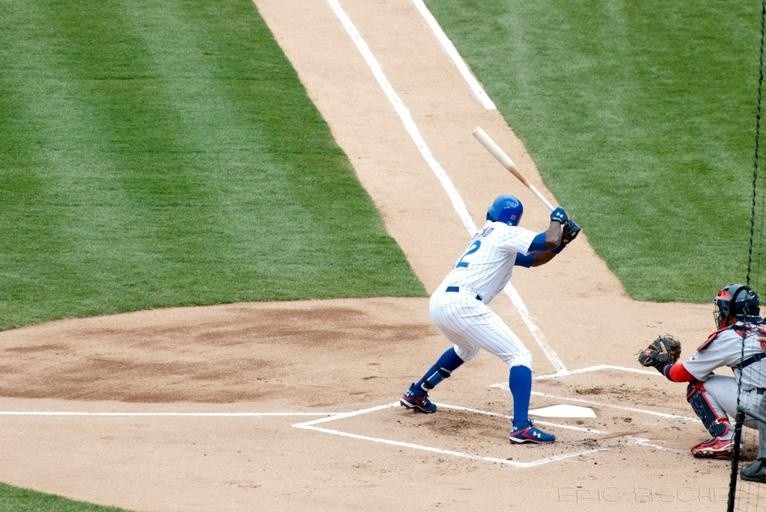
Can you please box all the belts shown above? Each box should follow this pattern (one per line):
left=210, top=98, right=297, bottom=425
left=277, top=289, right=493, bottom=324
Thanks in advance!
left=445, top=286, right=483, bottom=303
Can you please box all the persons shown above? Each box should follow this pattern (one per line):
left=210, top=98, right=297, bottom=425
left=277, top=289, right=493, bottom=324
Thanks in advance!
left=739, top=324, right=765, bottom=483
left=638, top=284, right=766, bottom=458
left=399, top=197, right=580, bottom=444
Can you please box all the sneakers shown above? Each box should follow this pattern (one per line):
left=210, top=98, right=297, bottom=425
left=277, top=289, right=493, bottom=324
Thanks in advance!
left=740, top=457, right=766, bottom=483
left=691, top=431, right=746, bottom=459
left=508, top=419, right=555, bottom=444
left=400, top=381, right=438, bottom=414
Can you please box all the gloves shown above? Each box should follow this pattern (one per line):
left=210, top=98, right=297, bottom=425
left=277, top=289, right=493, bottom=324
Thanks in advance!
left=550, top=206, right=568, bottom=225
left=563, top=220, right=580, bottom=241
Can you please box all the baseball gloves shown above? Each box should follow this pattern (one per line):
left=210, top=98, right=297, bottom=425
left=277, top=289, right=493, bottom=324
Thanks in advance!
left=639, top=337, right=680, bottom=367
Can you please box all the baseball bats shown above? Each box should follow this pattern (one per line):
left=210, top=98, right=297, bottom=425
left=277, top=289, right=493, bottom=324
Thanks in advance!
left=473, top=126, right=576, bottom=236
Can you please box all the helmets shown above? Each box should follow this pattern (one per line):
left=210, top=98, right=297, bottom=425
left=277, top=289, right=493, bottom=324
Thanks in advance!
left=486, top=195, right=524, bottom=226
left=711, top=283, right=760, bottom=330
